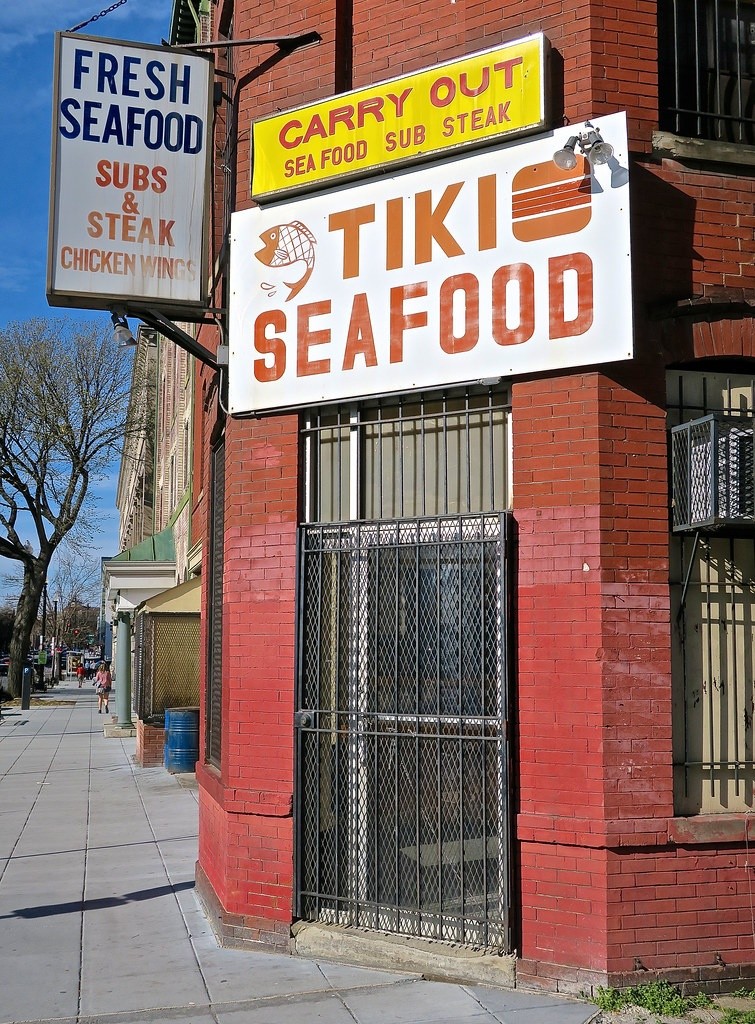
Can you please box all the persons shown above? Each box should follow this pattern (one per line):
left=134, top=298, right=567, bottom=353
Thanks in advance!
left=76, top=660, right=95, bottom=688
left=95, top=664, right=111, bottom=714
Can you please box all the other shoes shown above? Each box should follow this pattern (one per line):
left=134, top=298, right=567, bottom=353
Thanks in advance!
left=105, top=705, right=109, bottom=713
left=98, top=710, right=101, bottom=713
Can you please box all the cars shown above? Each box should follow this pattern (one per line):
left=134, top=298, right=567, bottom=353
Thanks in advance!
left=0, top=646, right=67, bottom=676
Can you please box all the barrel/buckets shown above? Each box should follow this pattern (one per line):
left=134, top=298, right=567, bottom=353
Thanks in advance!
left=163, top=707, right=200, bottom=774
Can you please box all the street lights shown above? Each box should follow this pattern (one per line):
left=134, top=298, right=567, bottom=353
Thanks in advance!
left=35, top=576, right=49, bottom=691
left=49, top=592, right=59, bottom=683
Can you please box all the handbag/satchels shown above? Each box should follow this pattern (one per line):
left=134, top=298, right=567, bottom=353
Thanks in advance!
left=96, top=688, right=104, bottom=695
